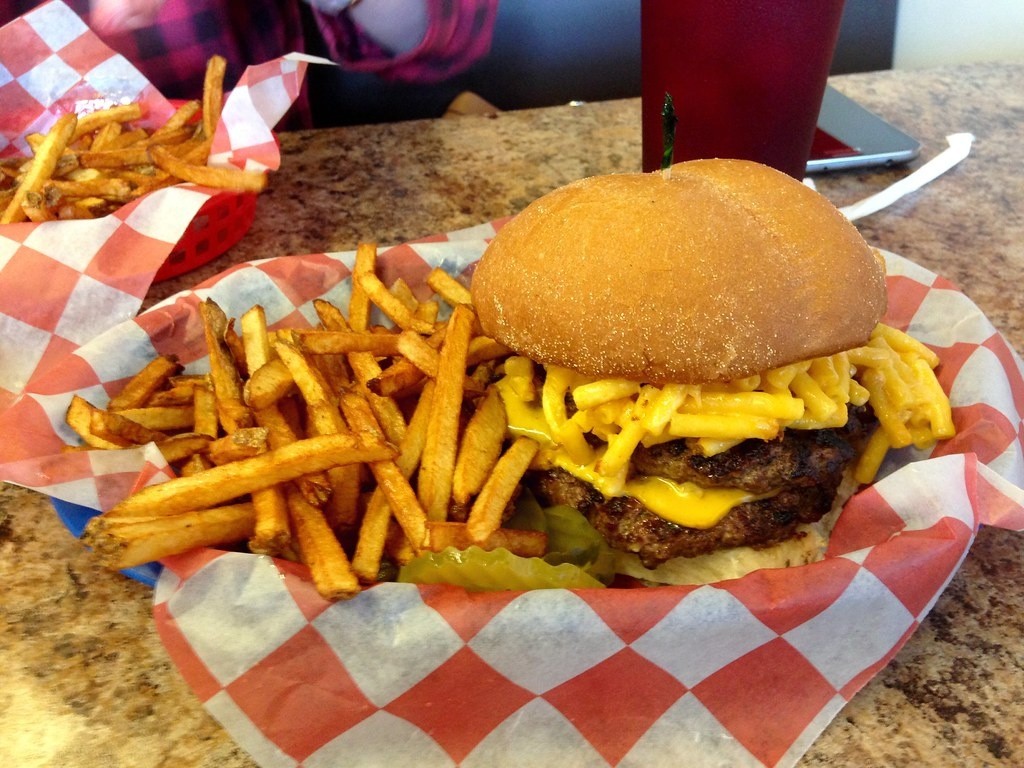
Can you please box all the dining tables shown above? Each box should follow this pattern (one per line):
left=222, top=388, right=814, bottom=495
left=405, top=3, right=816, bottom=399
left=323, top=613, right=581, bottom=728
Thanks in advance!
left=0, top=51, right=1024, bottom=768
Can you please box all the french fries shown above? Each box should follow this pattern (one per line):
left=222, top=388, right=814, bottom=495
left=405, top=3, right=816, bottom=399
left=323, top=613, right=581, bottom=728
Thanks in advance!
left=65, top=241, right=542, bottom=601
left=0, top=54, right=269, bottom=222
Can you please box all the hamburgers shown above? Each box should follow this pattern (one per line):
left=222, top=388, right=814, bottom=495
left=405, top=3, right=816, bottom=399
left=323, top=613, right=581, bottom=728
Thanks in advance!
left=472, top=158, right=954, bottom=586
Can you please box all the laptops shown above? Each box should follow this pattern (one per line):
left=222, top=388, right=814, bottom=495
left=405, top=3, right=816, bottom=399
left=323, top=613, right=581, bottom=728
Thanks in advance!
left=804, top=82, right=925, bottom=174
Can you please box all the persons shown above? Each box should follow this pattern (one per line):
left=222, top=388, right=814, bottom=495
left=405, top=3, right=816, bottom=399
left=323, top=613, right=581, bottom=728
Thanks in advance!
left=85, top=0, right=517, bottom=135
